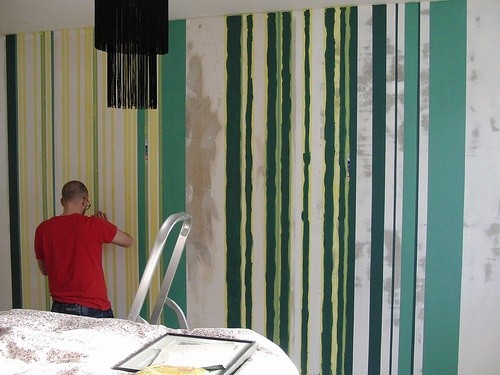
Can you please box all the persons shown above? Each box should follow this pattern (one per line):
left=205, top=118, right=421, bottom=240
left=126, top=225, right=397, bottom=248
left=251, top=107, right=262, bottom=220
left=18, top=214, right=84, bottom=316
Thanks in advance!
left=34, top=180, right=134, bottom=319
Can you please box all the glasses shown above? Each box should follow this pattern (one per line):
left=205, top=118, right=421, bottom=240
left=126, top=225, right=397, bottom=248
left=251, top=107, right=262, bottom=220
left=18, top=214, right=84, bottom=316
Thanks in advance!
left=86, top=199, right=91, bottom=210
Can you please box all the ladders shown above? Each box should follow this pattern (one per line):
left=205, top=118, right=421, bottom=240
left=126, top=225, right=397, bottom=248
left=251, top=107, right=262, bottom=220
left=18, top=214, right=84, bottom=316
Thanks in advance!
left=126, top=212, right=193, bottom=329
